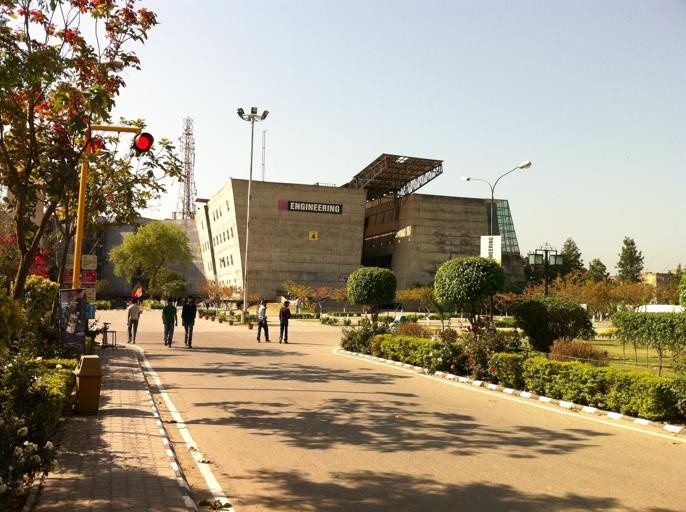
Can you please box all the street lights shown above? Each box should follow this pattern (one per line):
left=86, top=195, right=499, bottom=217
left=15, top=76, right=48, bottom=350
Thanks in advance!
left=528, top=249, right=564, bottom=296
left=236, top=106, right=269, bottom=323
left=466, top=160, right=531, bottom=323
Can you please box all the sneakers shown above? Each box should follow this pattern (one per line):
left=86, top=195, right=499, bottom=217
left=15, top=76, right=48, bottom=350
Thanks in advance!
left=128, top=340, right=135, bottom=344
left=184, top=342, right=192, bottom=348
left=257, top=339, right=288, bottom=343
left=164, top=341, right=171, bottom=348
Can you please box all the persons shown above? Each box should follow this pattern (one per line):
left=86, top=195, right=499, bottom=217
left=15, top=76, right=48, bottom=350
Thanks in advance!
left=162, top=298, right=178, bottom=347
left=126, top=298, right=140, bottom=343
left=257, top=301, right=271, bottom=343
left=278, top=301, right=291, bottom=343
left=182, top=296, right=196, bottom=348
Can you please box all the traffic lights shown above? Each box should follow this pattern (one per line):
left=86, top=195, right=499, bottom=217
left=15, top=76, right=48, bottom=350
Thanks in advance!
left=133, top=132, right=154, bottom=152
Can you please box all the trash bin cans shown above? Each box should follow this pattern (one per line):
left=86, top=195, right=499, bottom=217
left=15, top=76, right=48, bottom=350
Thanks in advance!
left=73, top=355, right=104, bottom=416
